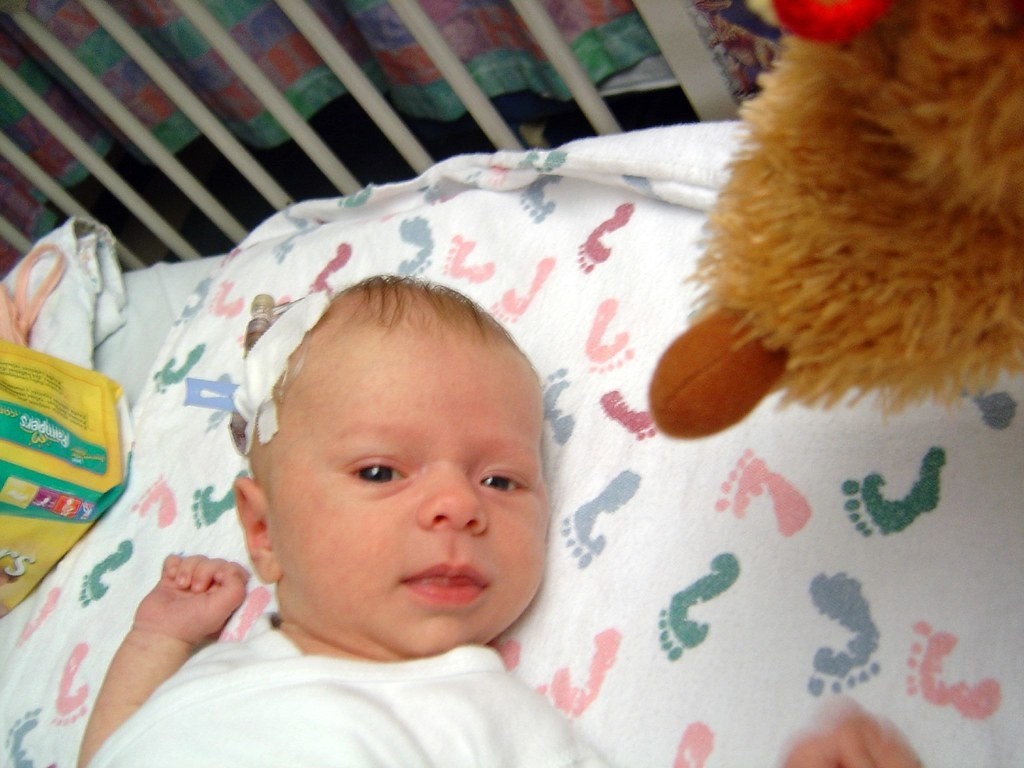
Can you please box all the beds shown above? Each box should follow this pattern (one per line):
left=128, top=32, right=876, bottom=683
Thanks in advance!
left=0, top=1, right=1024, bottom=768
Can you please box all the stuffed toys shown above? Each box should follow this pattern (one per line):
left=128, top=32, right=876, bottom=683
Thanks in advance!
left=649, top=1, right=1023, bottom=439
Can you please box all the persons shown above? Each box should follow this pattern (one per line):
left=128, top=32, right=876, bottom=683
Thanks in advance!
left=78, top=276, right=924, bottom=767
